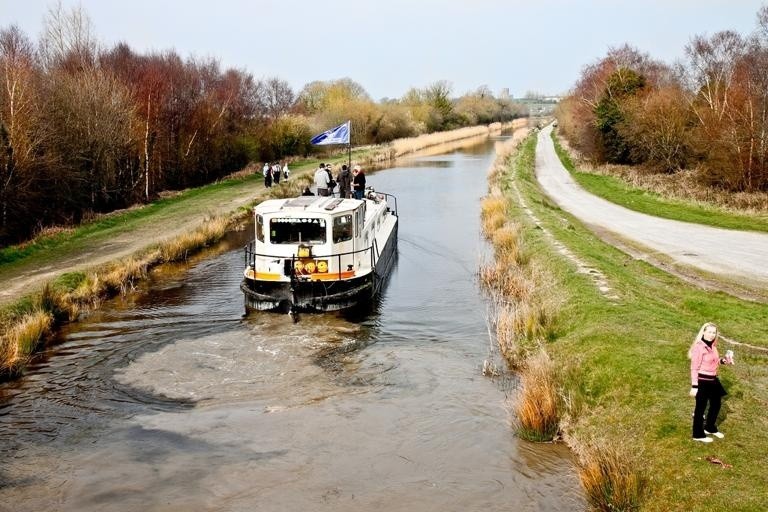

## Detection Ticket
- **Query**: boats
[240,120,398,315]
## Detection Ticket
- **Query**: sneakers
[704,429,724,439]
[693,436,713,443]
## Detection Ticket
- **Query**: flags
[310,121,350,145]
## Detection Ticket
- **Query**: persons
[273,161,282,184]
[263,161,273,188]
[302,185,315,197]
[314,162,366,199]
[690,321,737,446]
[283,161,290,182]
[270,161,276,180]
